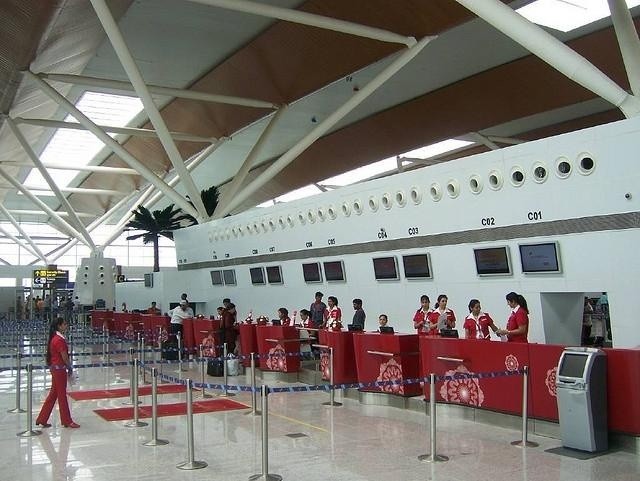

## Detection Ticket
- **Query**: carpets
[93,396,252,423]
[66,383,201,401]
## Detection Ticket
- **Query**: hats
[179,300,188,305]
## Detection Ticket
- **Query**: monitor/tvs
[518,241,562,275]
[348,324,363,331]
[556,350,593,383]
[472,245,513,277]
[372,255,400,281]
[266,265,284,285]
[271,319,281,325]
[323,259,347,284]
[210,270,224,285]
[210,316,214,319]
[144,274,154,288]
[440,328,459,338]
[402,252,433,280]
[379,326,393,332]
[301,261,324,284]
[249,266,266,286]
[223,269,237,285]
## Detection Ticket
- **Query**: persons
[34,318,81,429]
[146,293,366,361]
[413,295,435,336]
[376,314,391,332]
[495,292,529,343]
[579,292,613,349]
[34,427,73,481]
[16,291,82,326]
[428,294,456,336]
[462,299,504,341]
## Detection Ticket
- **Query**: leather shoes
[64,422,80,428]
[36,421,51,428]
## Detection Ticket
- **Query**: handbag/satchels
[227,353,239,376]
[207,359,223,376]
[161,340,184,360]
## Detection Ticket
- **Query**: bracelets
[494,329,499,335]
[507,330,511,335]
